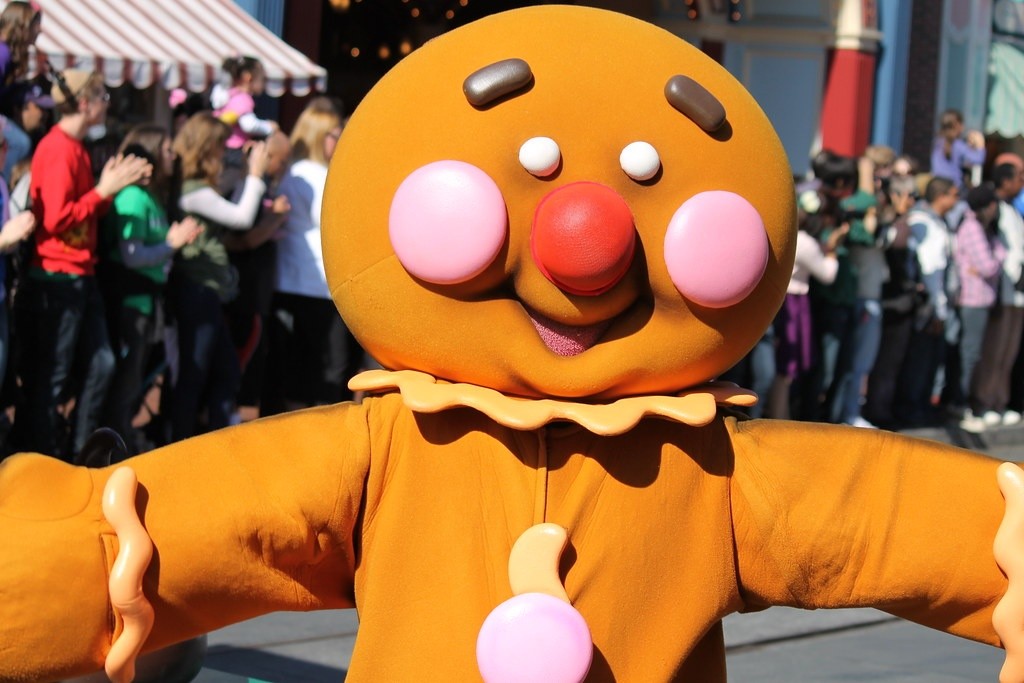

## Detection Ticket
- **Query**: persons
[761,109,1024,434]
[0,0,349,463]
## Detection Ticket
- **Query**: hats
[51,67,90,104]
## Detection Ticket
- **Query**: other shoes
[851,417,877,430]
[1002,409,1021,426]
[957,409,986,433]
[980,410,1000,425]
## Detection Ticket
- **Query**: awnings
[985,39,1024,137]
[0,0,328,98]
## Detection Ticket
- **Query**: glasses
[101,91,112,104]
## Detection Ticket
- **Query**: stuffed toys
[0,4,1024,683]
